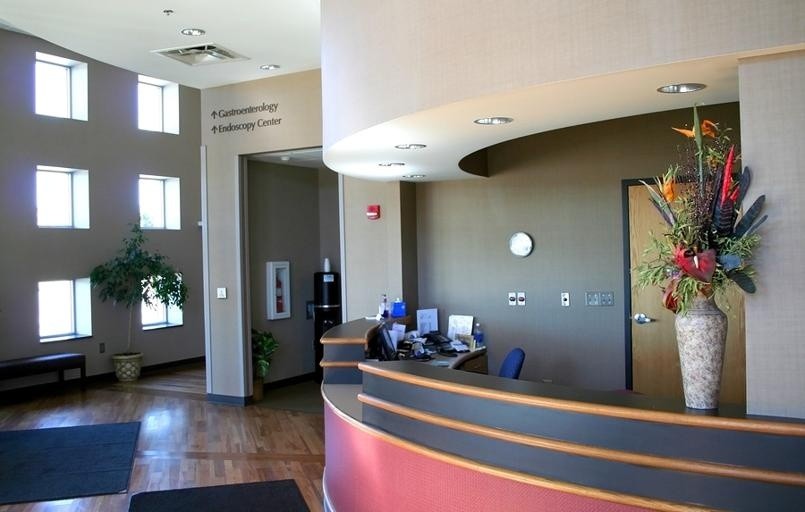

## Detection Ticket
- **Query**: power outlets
[216,287,226,299]
[600,291,613,305]
[586,292,600,306]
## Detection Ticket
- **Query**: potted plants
[90,223,189,382]
[250,326,277,401]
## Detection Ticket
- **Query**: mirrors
[510,233,533,257]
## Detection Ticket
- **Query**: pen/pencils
[383,293,387,312]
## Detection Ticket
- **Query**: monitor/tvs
[376,323,397,360]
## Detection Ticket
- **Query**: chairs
[500,348,525,381]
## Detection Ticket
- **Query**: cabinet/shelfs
[321,319,805,510]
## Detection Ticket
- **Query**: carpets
[128,480,310,512]
[0,421,141,504]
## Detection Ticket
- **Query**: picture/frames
[413,308,441,335]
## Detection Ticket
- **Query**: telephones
[450,340,470,353]
[423,332,459,358]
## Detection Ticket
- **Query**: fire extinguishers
[276,271,283,312]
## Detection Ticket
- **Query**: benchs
[0,352,85,406]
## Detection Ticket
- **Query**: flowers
[631,103,769,311]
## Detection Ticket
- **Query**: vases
[677,284,727,407]
